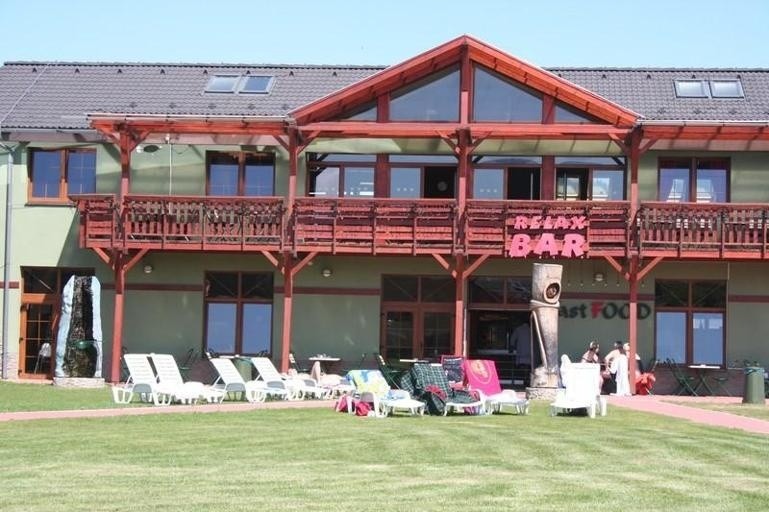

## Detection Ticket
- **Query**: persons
[621,342,644,374]
[510,315,538,390]
[599,340,627,395]
[579,340,600,363]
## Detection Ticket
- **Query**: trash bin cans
[742,367,765,405]
[233,357,252,382]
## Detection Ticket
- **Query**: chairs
[331,347,606,418]
[643,357,740,395]
[111,349,344,407]
